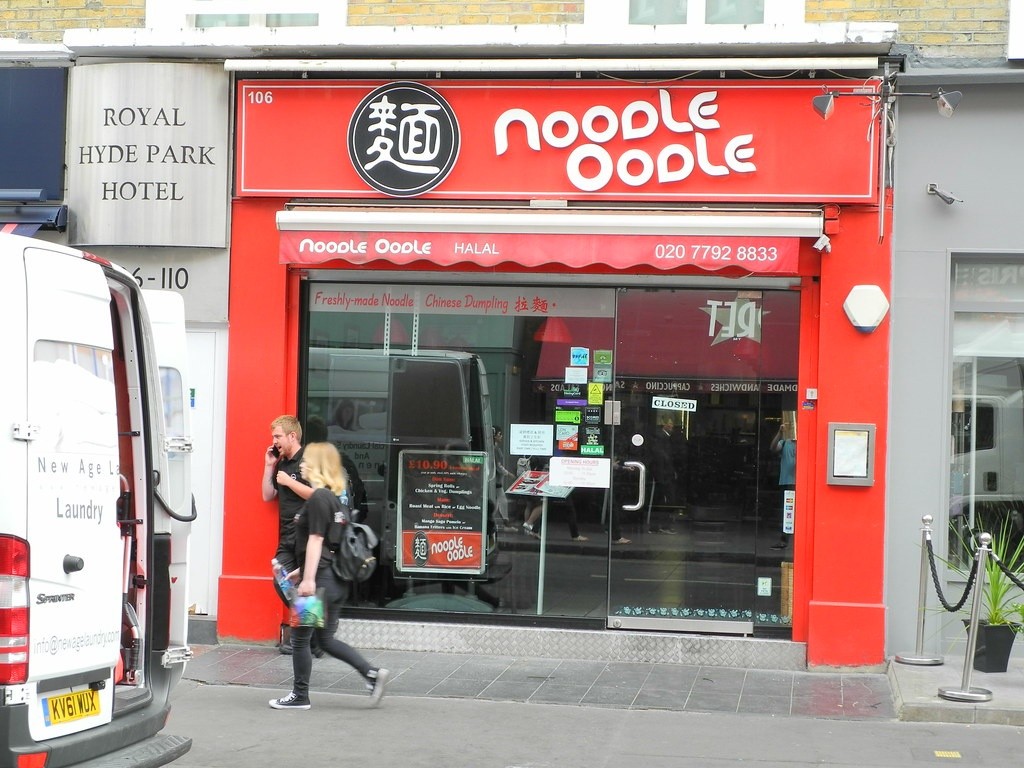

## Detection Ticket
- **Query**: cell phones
[271,445,280,458]
[785,423,794,431]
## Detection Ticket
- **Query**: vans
[307,342,513,598]
[0,230,202,768]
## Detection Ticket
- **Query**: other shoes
[526,529,541,540]
[611,537,632,545]
[648,528,661,534]
[522,522,538,533]
[573,534,590,542]
[659,528,678,535]
[769,543,789,550]
[278,638,293,655]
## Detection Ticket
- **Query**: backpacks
[331,498,380,584]
[517,455,533,477]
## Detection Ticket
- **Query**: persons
[267,442,389,709]
[261,416,332,658]
[304,400,357,442]
[442,414,796,610]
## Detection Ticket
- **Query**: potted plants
[919,511,1024,674]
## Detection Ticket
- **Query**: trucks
[952,389,1024,565]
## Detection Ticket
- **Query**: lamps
[843,284,890,332]
[892,87,962,118]
[813,92,882,119]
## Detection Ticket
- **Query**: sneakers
[269,691,311,709]
[366,668,391,709]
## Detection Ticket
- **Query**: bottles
[271,558,296,601]
[339,490,348,505]
